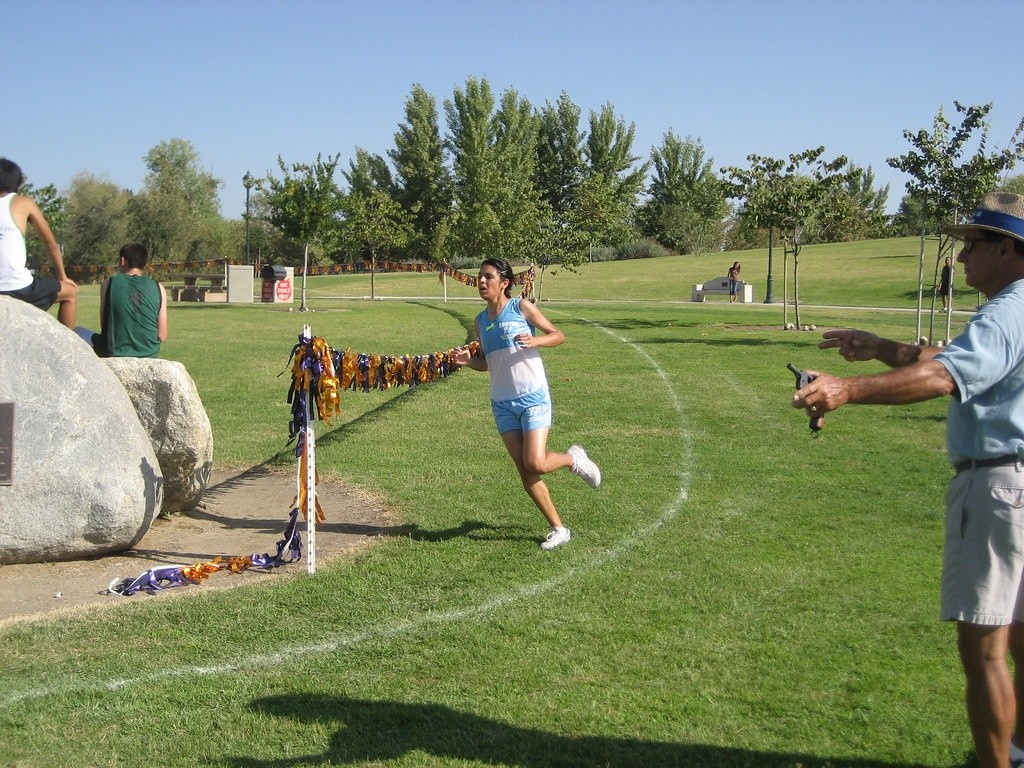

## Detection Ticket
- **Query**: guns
[787,363,826,432]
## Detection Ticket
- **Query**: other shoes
[939,308,948,313]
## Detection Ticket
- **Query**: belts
[956,455,1024,472]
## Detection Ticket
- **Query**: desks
[166,273,225,301]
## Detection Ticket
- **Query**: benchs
[164,286,227,301]
[692,277,753,303]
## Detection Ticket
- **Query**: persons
[0,158,79,331]
[72,242,167,358]
[449,256,601,549]
[791,192,1024,768]
[938,257,957,314]
[727,262,740,303]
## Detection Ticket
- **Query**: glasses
[964,236,1003,251]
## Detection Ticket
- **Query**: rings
[810,403,817,414]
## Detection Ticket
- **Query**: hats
[944,192,1024,242]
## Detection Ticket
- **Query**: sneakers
[540,528,570,551]
[567,444,601,489]
